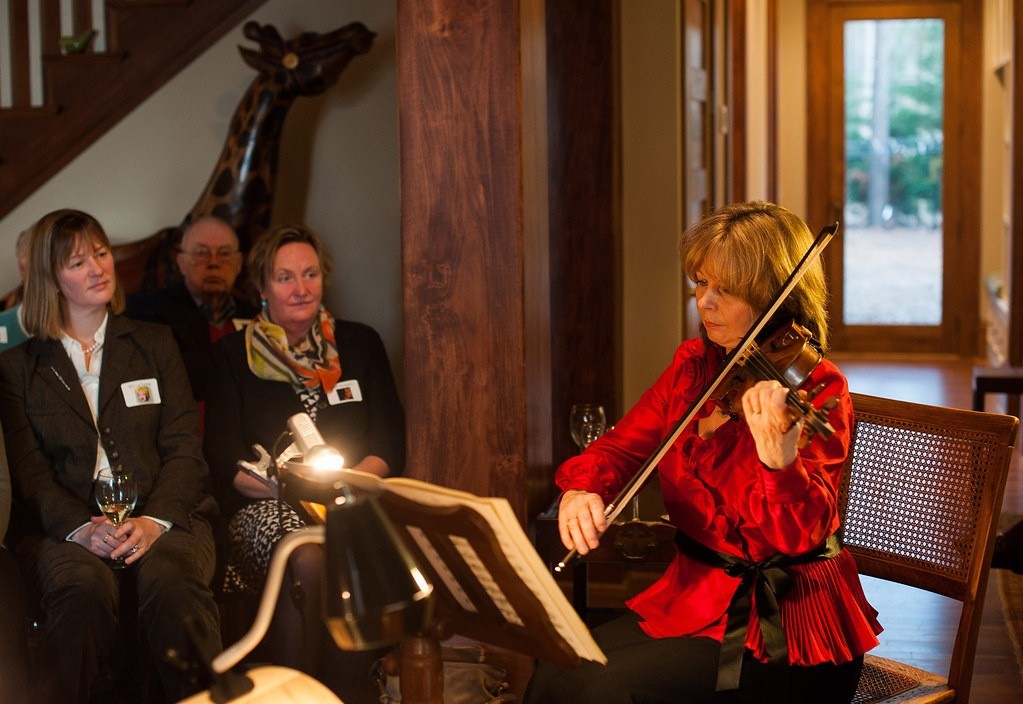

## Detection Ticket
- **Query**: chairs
[972,365,1023,434]
[838,393,1021,704]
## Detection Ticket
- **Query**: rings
[131,545,138,552]
[103,535,108,543]
[567,517,577,522]
[753,411,760,414]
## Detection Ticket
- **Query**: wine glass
[95,466,138,570]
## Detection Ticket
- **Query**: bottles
[398,620,446,704]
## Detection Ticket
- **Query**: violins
[708,310,841,443]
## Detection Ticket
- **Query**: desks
[533,478,672,620]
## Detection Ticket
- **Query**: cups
[570,403,606,454]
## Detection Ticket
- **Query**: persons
[128,216,260,444]
[205,222,407,680]
[0,209,222,704]
[527,203,885,704]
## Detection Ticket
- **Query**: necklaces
[84,343,98,353]
[717,410,727,417]
